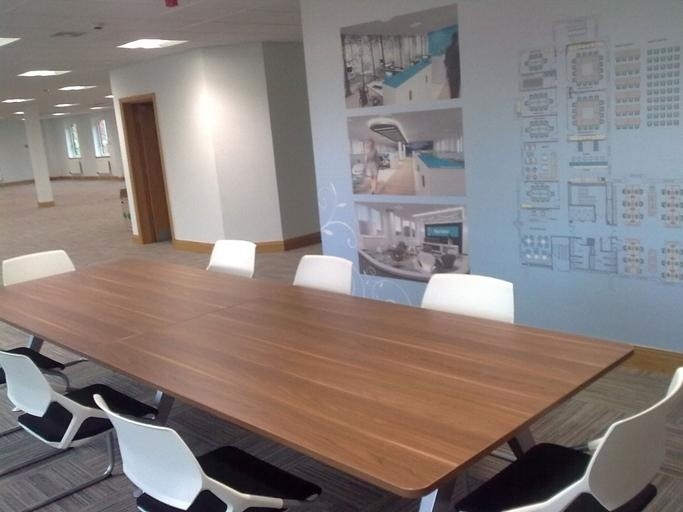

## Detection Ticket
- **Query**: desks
[1,256,633,511]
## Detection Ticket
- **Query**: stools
[1,347,73,438]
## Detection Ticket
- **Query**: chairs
[204,239,256,280]
[420,273,515,326]
[2,249,76,286]
[451,366,682,511]
[2,351,157,511]
[291,254,352,297]
[90,392,322,511]
